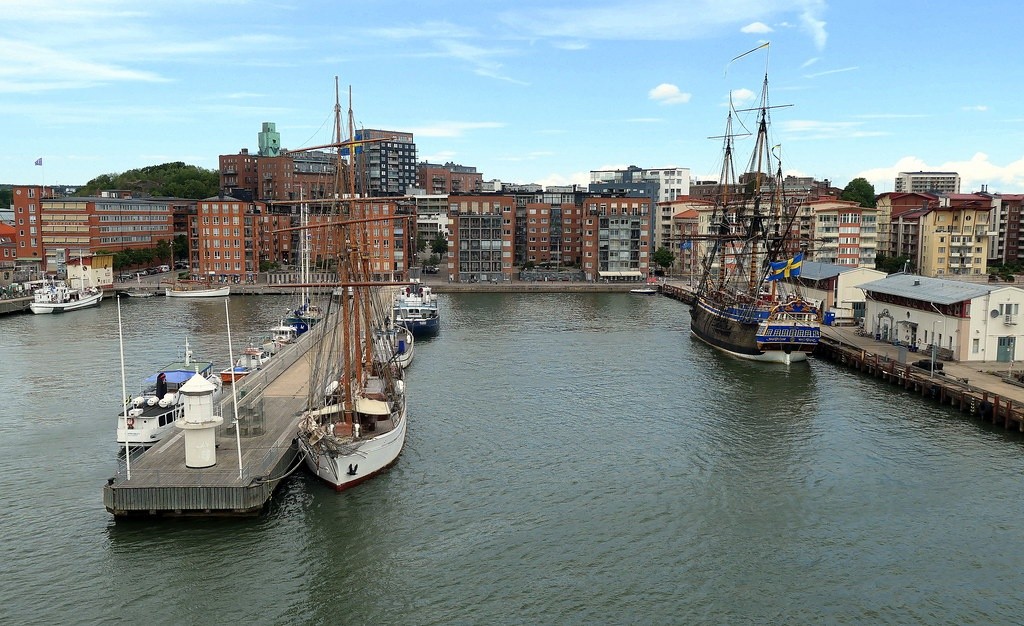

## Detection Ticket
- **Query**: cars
[113,264,170,280]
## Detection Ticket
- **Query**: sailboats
[261,76,426,493]
[688,39,825,367]
[164,241,231,298]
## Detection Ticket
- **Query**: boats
[384,276,441,336]
[27,249,105,315]
[630,287,657,294]
[219,185,353,386]
[121,289,154,298]
[360,269,416,369]
[115,338,224,447]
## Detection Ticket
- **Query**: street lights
[864,292,878,341]
[903,259,911,272]
[838,298,851,346]
[930,320,943,378]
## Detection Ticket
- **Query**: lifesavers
[978,400,992,416]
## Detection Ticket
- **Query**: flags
[35,158,42,166]
[767,252,804,281]
